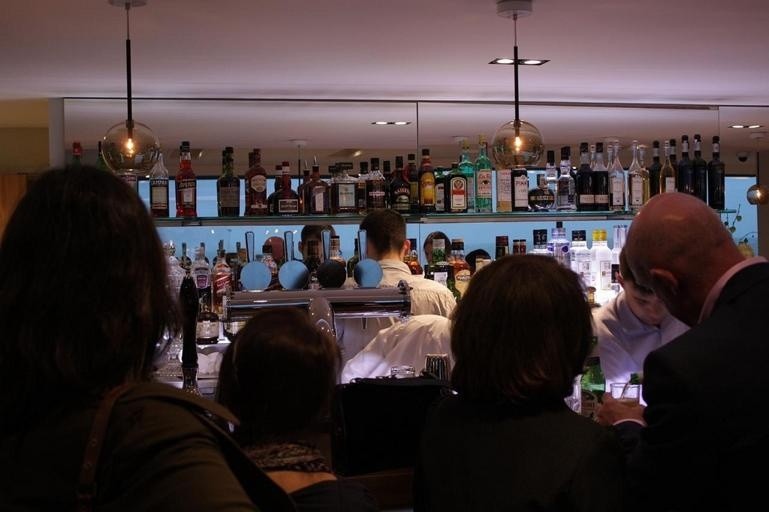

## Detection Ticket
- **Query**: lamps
[493,0,543,168]
[102,0,159,177]
[747,132,769,205]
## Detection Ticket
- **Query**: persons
[594,192,769,511]
[0,162,297,512]
[597,244,692,407]
[211,307,377,510]
[413,254,628,510]
[296,208,491,385]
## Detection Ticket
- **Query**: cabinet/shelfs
[138,209,740,397]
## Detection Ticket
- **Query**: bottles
[458,141,493,214]
[406,148,433,213]
[175,140,198,216]
[527,173,555,211]
[268,161,301,215]
[149,134,169,217]
[244,147,268,217]
[298,156,411,215]
[150,221,633,346]
[496,154,528,212]
[216,147,241,217]
[581,337,607,421]
[96,139,109,170]
[72,139,84,163]
[433,162,468,213]
[545,132,724,213]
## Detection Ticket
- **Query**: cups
[391,364,415,379]
[610,382,642,407]
